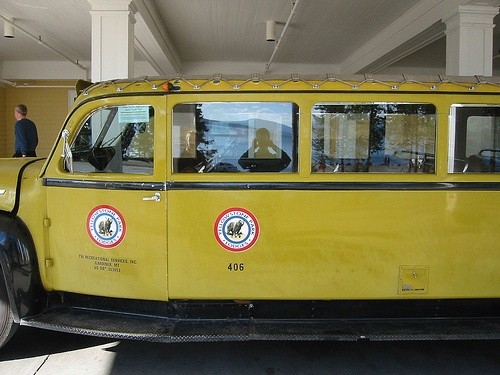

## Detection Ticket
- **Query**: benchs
[309,148,500,173]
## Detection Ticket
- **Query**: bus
[0,74,500,350]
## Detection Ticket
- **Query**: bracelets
[22,154,27,156]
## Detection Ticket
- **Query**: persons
[177,131,211,173]
[238,129,290,172]
[10,103,39,159]
